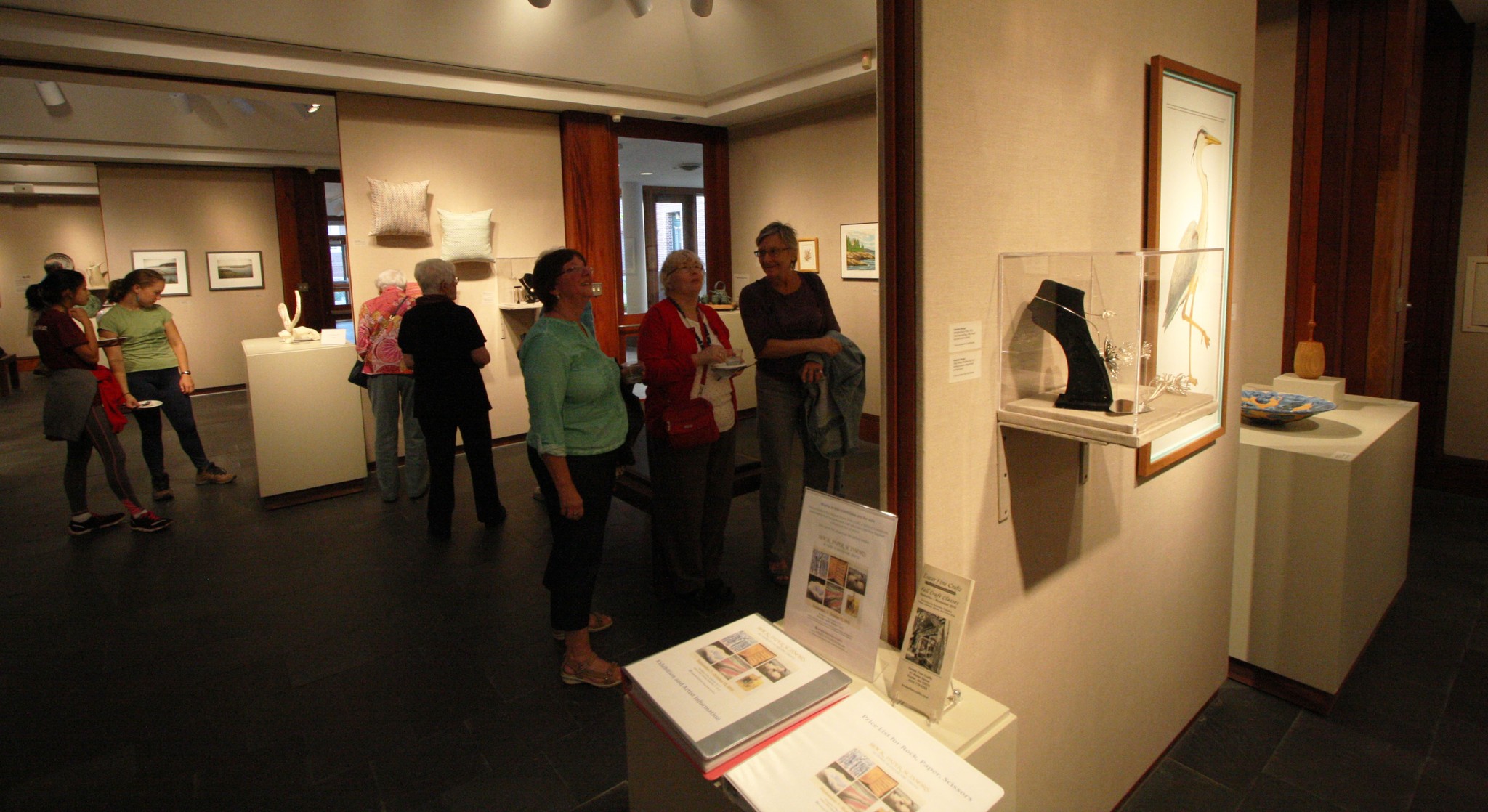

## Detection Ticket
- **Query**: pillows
[435,208,496,265]
[367,176,432,238]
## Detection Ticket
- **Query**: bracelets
[181,371,191,375]
[124,392,130,395]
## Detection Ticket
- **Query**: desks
[610,446,765,517]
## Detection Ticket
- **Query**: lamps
[303,103,321,113]
[625,0,653,18]
[690,0,714,17]
[171,92,193,114]
[528,0,550,8]
[36,80,64,106]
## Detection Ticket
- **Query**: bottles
[513,286,522,304]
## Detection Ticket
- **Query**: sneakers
[196,460,236,485]
[151,484,174,500]
[128,510,172,532]
[70,511,125,535]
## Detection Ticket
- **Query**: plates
[97,337,128,344]
[138,400,163,408]
[43,253,74,274]
[713,362,747,370]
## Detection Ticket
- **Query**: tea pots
[709,281,727,301]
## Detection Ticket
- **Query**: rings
[720,353,722,358]
[574,513,578,515]
[814,369,819,373]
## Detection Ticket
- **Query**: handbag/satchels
[662,397,720,446]
[347,357,369,389]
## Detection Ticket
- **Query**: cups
[621,361,646,383]
[1293,340,1325,380]
[711,295,721,304]
[701,295,709,304]
[721,296,732,304]
[724,349,742,365]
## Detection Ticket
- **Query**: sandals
[553,611,613,641]
[560,653,623,688]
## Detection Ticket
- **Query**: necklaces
[669,297,711,351]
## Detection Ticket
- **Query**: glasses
[558,266,594,278]
[754,248,790,258]
[450,277,459,284]
[666,263,703,277]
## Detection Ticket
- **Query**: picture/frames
[798,237,819,273]
[131,249,191,297]
[205,250,265,291]
[1137,56,1243,479]
[840,223,880,281]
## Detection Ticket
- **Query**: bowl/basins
[1240,390,1337,427]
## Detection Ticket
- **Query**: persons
[398,258,507,550]
[96,268,237,502]
[515,248,648,687]
[636,250,745,608]
[25,260,174,535]
[357,270,430,504]
[739,221,843,585]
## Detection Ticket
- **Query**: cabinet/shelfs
[1226,386,1421,713]
[240,336,369,509]
[626,616,1019,812]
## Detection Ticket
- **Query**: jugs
[86,262,106,287]
